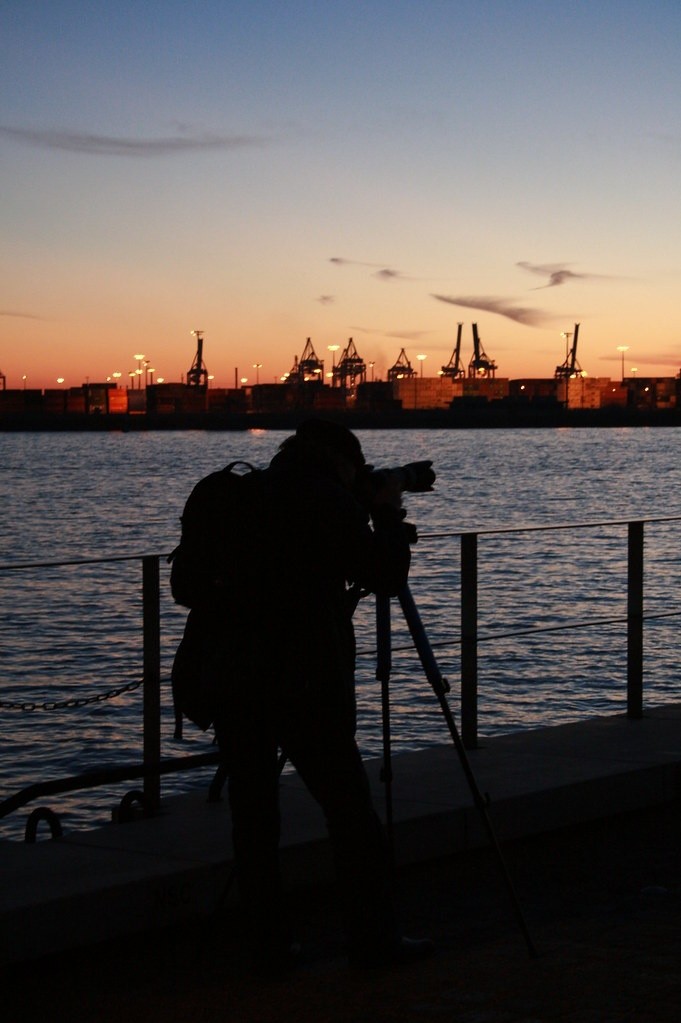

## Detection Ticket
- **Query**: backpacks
[167,462,318,783]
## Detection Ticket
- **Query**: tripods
[196,516,543,960]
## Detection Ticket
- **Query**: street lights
[112,371,121,383]
[253,364,262,384]
[22,375,27,389]
[147,368,155,384]
[368,362,375,382]
[134,354,145,389]
[143,361,150,385]
[617,346,630,380]
[328,345,339,366]
[561,332,573,367]
[129,372,136,389]
[417,354,426,378]
[631,367,638,378]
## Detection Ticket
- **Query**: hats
[297,418,357,465]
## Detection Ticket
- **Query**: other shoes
[349,937,433,968]
[257,959,297,984]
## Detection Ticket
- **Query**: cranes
[296,336,325,386]
[467,322,498,379]
[186,337,210,390]
[330,336,367,395]
[553,322,582,380]
[386,348,419,383]
[439,321,466,379]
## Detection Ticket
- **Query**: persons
[173,425,436,962]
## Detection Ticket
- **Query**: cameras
[360,459,437,518]
